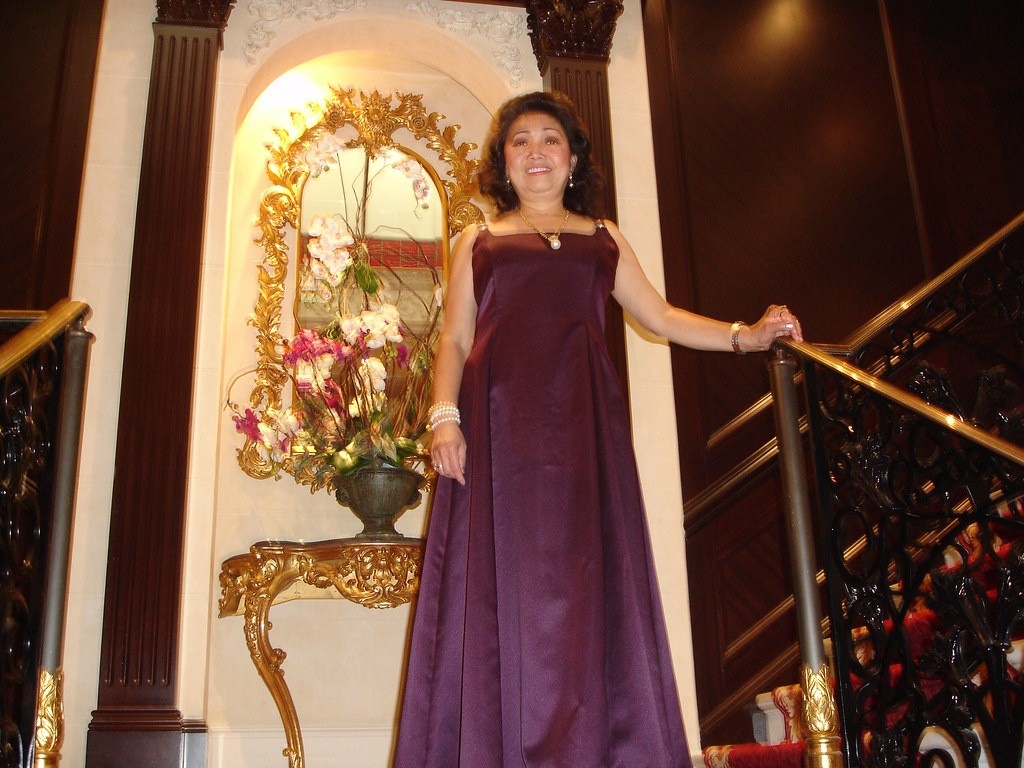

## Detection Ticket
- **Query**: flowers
[224,124,448,482]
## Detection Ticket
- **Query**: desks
[218,540,424,768]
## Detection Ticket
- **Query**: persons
[387,91,803,768]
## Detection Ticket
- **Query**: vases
[335,468,429,537]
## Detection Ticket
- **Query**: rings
[779,305,799,323]
[434,464,442,470]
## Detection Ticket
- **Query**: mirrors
[235,83,488,495]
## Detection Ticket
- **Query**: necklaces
[517,206,569,249]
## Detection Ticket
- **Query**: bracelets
[427,402,461,428]
[731,321,749,355]
